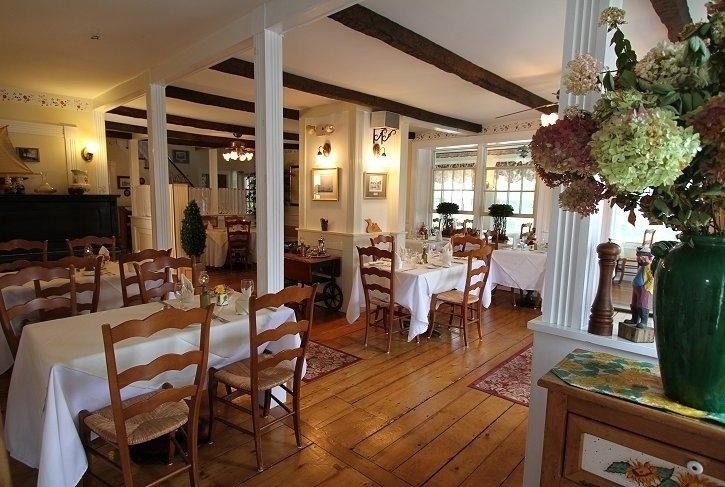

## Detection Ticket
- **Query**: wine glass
[241,279,255,295]
[198,271,210,285]
[173,283,190,302]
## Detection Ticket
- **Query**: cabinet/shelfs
[531,345,724,487]
[0,193,122,253]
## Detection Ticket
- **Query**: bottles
[34,167,55,193]
[586,238,619,337]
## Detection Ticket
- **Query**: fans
[493,89,559,119]
[194,130,257,152]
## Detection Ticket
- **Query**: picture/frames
[362,170,390,200]
[308,166,343,204]
[172,149,191,164]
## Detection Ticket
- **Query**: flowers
[525,0,725,236]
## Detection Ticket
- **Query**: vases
[0,168,90,194]
[648,233,725,411]
[116,173,130,191]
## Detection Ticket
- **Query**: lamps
[222,149,254,162]
[539,110,558,126]
[79,144,97,163]
[371,143,390,161]
[315,141,332,159]
[305,120,336,137]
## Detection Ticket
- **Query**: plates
[188,320,215,329]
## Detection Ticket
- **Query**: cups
[320,218,329,232]
[421,247,428,264]
[419,221,428,240]
